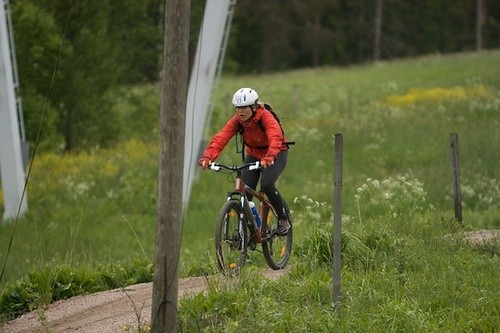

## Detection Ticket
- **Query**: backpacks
[235,104,284,150]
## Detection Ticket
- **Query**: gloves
[261,156,274,168]
[198,158,209,170]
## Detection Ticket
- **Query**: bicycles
[206,160,293,278]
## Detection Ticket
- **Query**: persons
[199,88,291,236]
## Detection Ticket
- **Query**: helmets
[232,88,259,107]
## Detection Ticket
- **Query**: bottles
[248,202,261,227]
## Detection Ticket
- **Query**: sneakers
[273,218,291,235]
